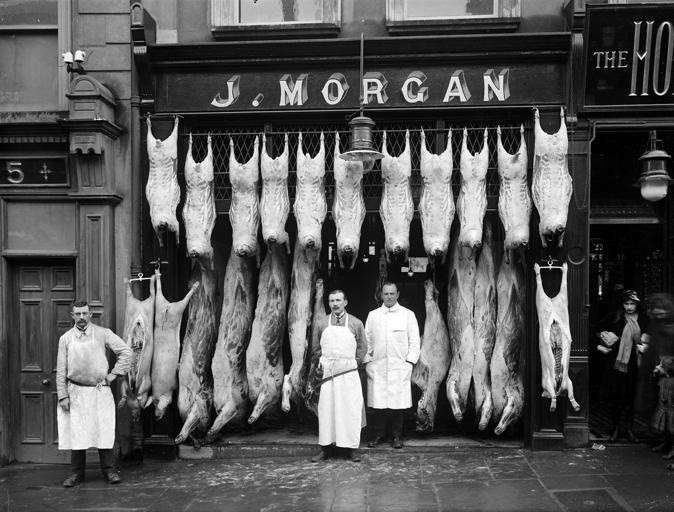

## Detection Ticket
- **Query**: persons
[596,289,649,445]
[645,292,673,460]
[311,290,366,462]
[363,282,420,450]
[55,301,133,488]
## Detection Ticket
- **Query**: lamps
[338,28,387,176]
[635,131,672,202]
[62,50,87,74]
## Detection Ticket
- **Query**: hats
[624,289,640,301]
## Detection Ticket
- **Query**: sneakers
[347,451,360,461]
[368,438,383,446]
[63,474,83,486]
[107,471,121,483]
[393,438,402,448]
[312,451,327,461]
